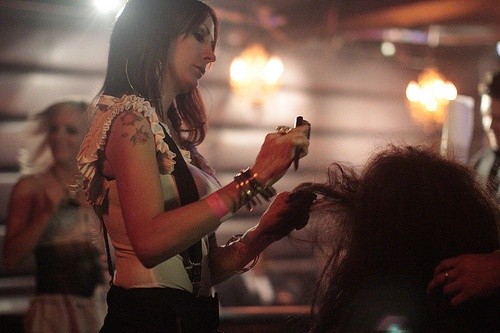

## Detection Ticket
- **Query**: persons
[85,0,315,333]
[290,69,500,333]
[0,99,134,333]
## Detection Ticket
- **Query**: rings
[444,272,449,281]
[276,126,292,134]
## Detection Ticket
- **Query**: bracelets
[201,169,277,221]
[223,234,260,274]
